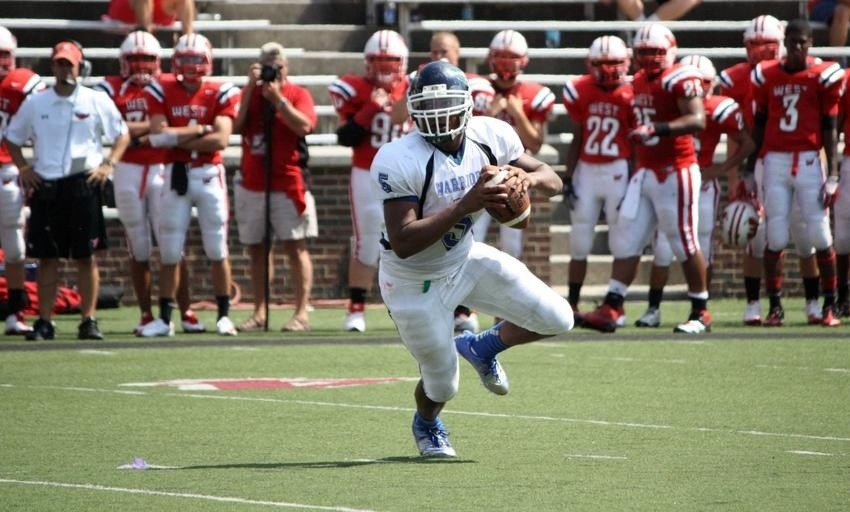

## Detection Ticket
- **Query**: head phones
[66,38,92,77]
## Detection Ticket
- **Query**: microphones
[60,78,86,87]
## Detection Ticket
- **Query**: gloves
[819,175,841,209]
[735,172,758,201]
[561,176,578,210]
[627,123,655,145]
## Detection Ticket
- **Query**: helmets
[120,31,162,78]
[406,62,473,145]
[743,15,784,66]
[364,30,409,86]
[173,34,213,85]
[632,23,678,75]
[680,55,716,102]
[486,30,529,82]
[0,25,17,76]
[721,201,758,248]
[589,35,631,88]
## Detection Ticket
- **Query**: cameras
[259,65,278,82]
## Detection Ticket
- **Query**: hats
[52,40,83,65]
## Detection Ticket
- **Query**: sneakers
[78,315,103,339]
[762,306,786,326]
[24,317,55,342]
[215,316,238,336]
[281,317,311,332]
[133,312,153,333]
[180,309,205,332]
[454,333,510,396]
[805,298,823,324]
[455,313,480,334]
[343,303,366,333]
[412,413,456,459]
[4,319,30,334]
[634,308,661,328]
[235,313,265,332]
[821,296,841,326]
[135,318,175,338]
[834,297,850,318]
[743,300,762,324]
[573,304,619,332]
[616,307,625,327]
[673,309,713,334]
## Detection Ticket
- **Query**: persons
[461,29,557,332]
[92,30,207,338]
[616,1,704,21]
[232,41,319,333]
[106,0,196,35]
[635,55,757,329]
[829,0,849,46]
[561,34,636,327]
[833,66,850,324]
[326,30,414,332]
[743,17,850,328]
[390,31,497,331]
[137,32,244,339]
[2,40,131,341]
[573,23,713,334]
[369,60,576,461]
[719,13,824,325]
[0,25,46,336]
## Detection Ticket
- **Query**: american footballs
[481,165,531,230]
